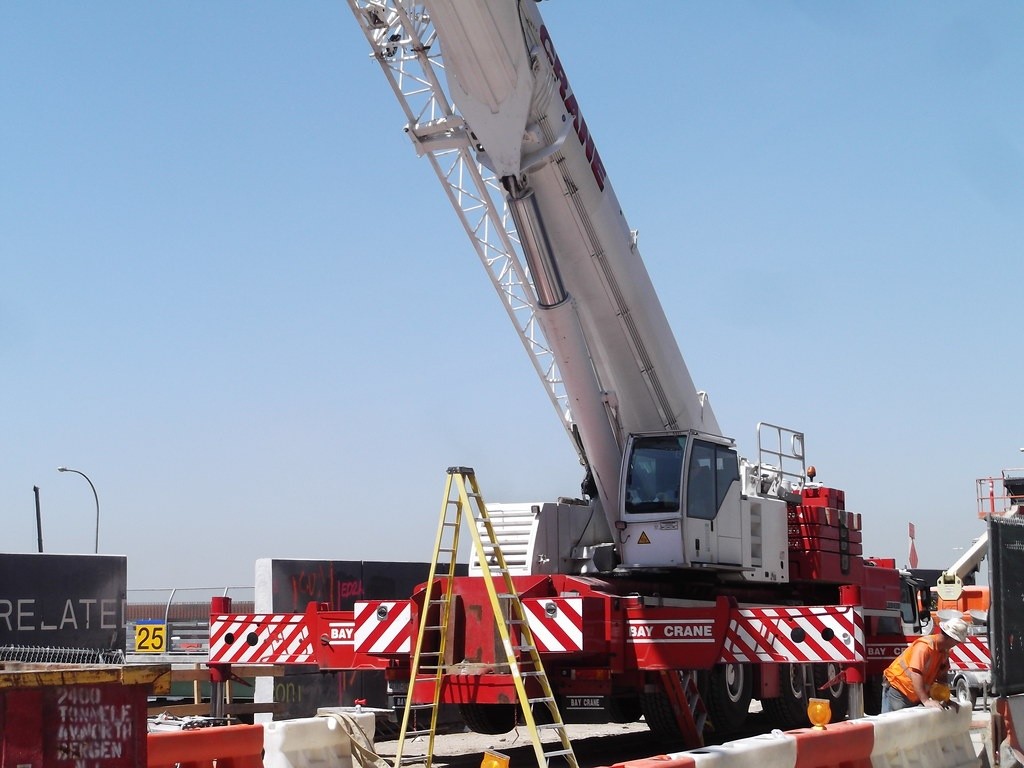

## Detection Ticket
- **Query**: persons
[881,616,967,714]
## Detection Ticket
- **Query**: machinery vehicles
[203,2,918,752]
[916,472,1024,712]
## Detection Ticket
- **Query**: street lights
[58,466,100,555]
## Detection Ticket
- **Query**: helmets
[939,618,968,643]
